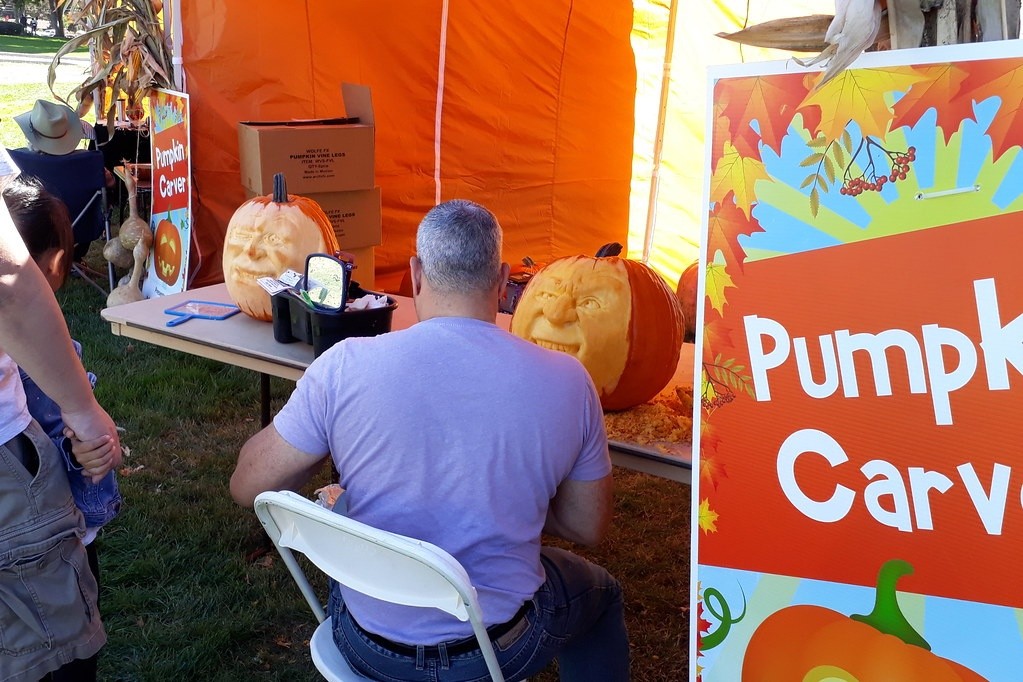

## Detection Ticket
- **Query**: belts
[347,600,537,658]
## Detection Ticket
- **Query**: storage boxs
[238,79,383,197]
[331,246,378,292]
[288,184,382,255]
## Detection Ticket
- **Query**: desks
[101,274,698,487]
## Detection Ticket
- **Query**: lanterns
[507,240,686,414]
[222,173,342,323]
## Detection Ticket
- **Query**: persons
[1,178,121,612]
[1,188,119,682]
[4,12,41,38]
[228,197,631,682]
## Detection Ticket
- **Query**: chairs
[248,488,506,682]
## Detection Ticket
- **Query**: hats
[13,99,84,155]
